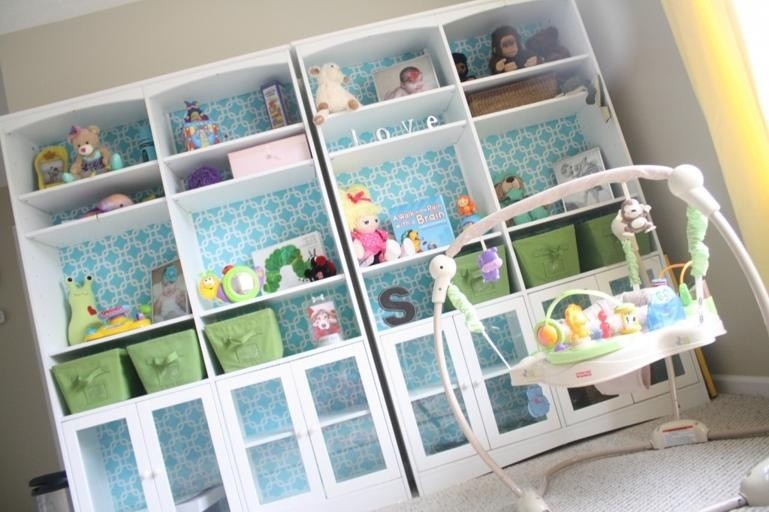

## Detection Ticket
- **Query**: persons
[48,166,59,184]
[384,66,428,101]
[153,264,188,323]
[402,230,420,254]
[561,156,605,208]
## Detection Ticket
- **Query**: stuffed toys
[494,173,549,227]
[188,165,221,189]
[307,61,361,126]
[559,73,597,104]
[452,52,476,82]
[621,199,656,234]
[488,26,541,73]
[67,274,104,346]
[337,184,416,269]
[477,247,502,284]
[198,264,266,310]
[82,192,135,217]
[181,100,221,152]
[456,195,482,233]
[525,27,572,65]
[304,255,337,282]
[61,124,123,183]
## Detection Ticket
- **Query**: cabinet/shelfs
[292,2,712,497]
[0,46,413,511]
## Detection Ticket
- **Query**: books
[251,231,327,296]
[551,146,615,213]
[387,193,455,255]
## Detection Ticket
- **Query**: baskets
[467,69,558,118]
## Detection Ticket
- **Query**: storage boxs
[51,347,128,414]
[583,211,651,268]
[203,307,285,374]
[126,328,204,394]
[511,225,582,288]
[453,243,511,305]
